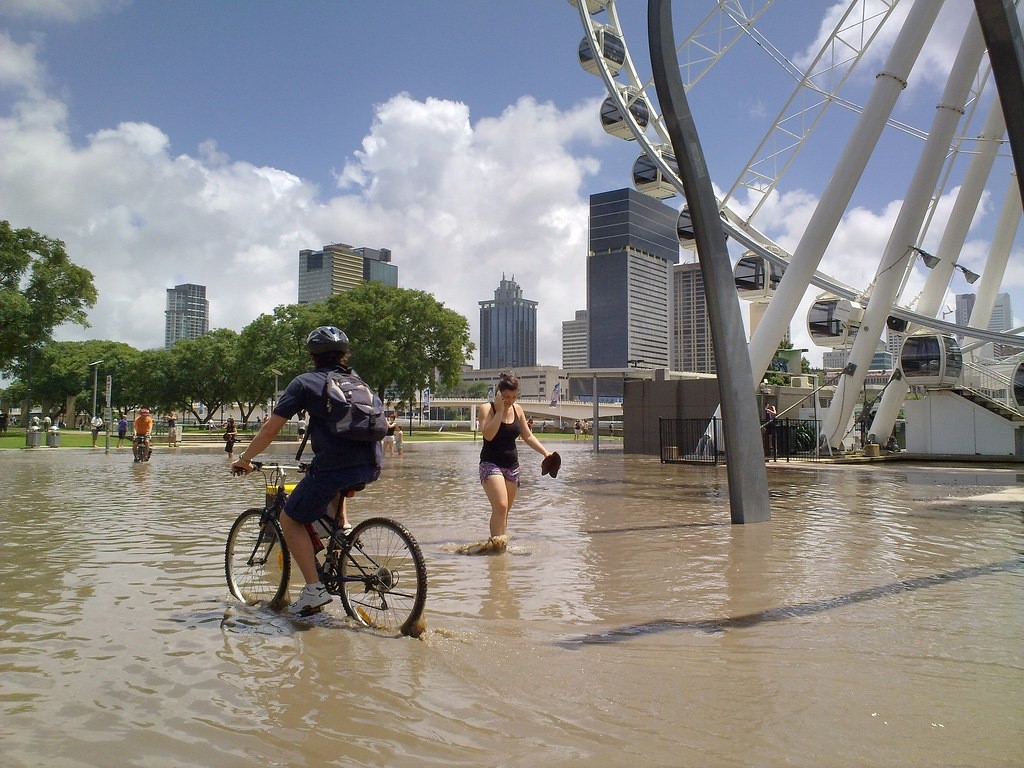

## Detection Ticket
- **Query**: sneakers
[289,581,334,614]
[325,524,354,545]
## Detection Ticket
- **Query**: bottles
[305,523,325,554]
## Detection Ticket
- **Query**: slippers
[542,452,561,479]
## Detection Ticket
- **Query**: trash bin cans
[26,426,41,446]
[46,426,61,446]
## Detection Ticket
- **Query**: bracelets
[239,451,251,464]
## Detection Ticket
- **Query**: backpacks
[316,369,386,441]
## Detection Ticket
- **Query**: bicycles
[223,459,427,635]
[132,434,154,462]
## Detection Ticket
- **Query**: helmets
[304,326,348,360]
[227,417,234,425]
[140,409,150,416]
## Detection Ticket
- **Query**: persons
[90,413,103,447]
[231,326,382,615]
[527,419,533,430]
[44,415,51,432]
[298,417,306,439]
[0,409,8,437]
[132,408,153,462]
[256,413,269,423]
[225,414,237,458]
[765,402,776,449]
[477,372,552,538]
[32,415,39,426]
[383,415,403,457]
[117,415,127,448]
[574,419,589,440]
[167,411,178,447]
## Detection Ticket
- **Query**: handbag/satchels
[266,485,352,585]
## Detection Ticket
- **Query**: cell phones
[496,390,502,397]
